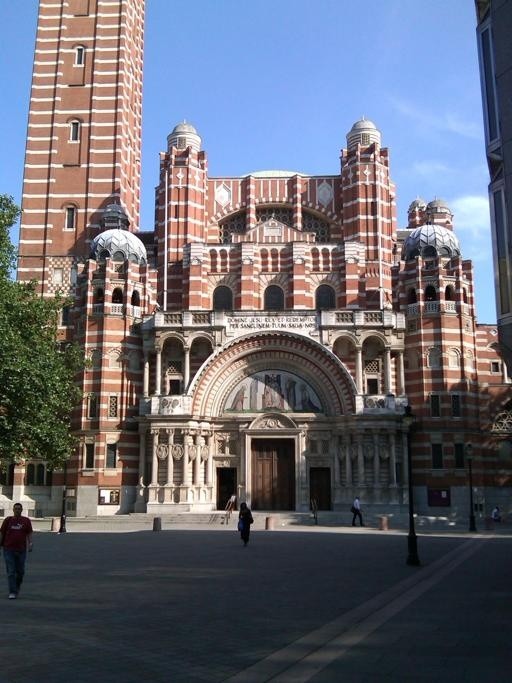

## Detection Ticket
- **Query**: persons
[224,374,318,412]
[0,502,36,600]
[491,505,502,522]
[237,502,254,547]
[350,495,364,526]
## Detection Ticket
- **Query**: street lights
[399,406,421,564]
[60,448,71,532]
[466,444,477,531]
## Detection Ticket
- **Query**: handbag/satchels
[237,518,244,532]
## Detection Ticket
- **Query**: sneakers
[8,592,18,599]
